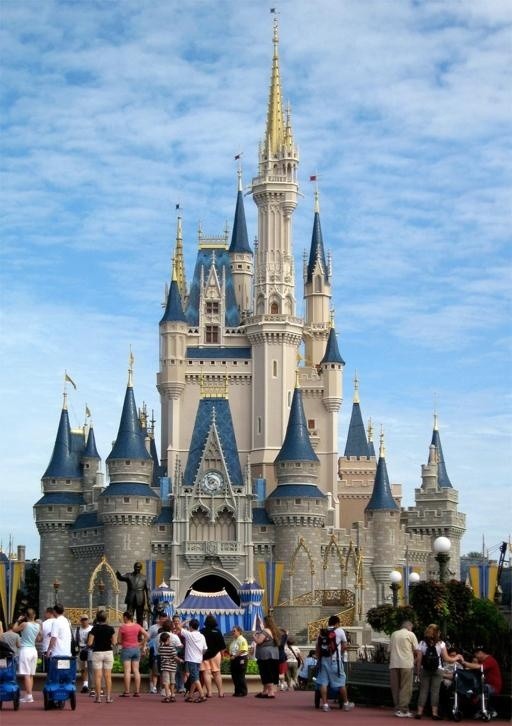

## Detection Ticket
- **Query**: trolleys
[308,651,346,709]
[0,653,22,711]
[448,662,490,723]
[42,656,79,712]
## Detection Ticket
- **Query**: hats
[79,613,89,621]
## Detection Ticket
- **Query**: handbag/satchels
[71,638,80,657]
[296,656,304,668]
[79,649,89,661]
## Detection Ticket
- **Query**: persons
[199,616,226,698]
[253,615,281,698]
[1,604,189,702]
[317,616,355,712]
[251,628,318,691]
[178,619,208,703]
[229,626,248,696]
[387,621,502,720]
[116,562,151,642]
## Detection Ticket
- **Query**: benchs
[311,660,444,714]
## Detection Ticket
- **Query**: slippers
[206,691,226,698]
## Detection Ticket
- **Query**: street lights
[53,579,61,607]
[98,579,106,612]
[407,571,420,585]
[389,571,402,608]
[433,537,452,584]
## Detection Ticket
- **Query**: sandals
[170,696,177,703]
[160,697,171,703]
[185,697,194,702]
[133,692,141,697]
[194,696,208,703]
[118,691,131,697]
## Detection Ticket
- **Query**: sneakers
[158,687,167,697]
[150,686,158,694]
[100,690,105,696]
[473,710,499,721]
[19,694,34,703]
[94,697,102,703]
[232,692,276,698]
[322,705,331,712]
[393,709,442,721]
[106,697,114,703]
[88,691,96,697]
[343,701,355,711]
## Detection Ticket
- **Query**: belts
[78,644,88,649]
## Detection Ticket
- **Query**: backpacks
[317,626,339,657]
[422,638,438,672]
